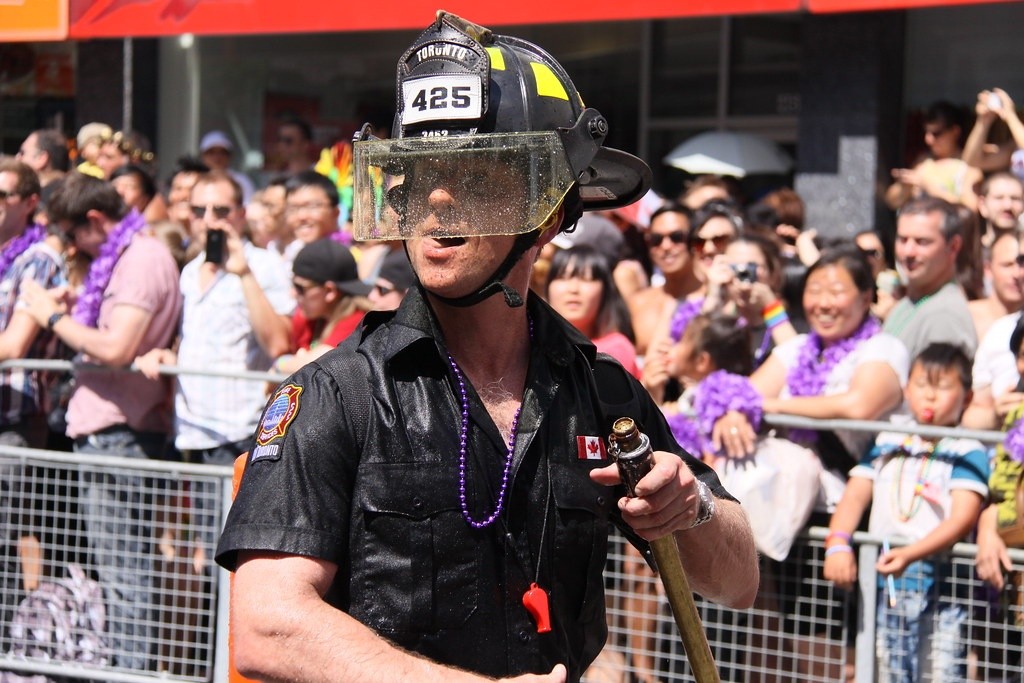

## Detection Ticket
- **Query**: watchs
[690,477,715,528]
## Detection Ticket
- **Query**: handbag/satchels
[712,429,823,561]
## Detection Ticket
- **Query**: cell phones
[988,94,1002,112]
[206,229,224,264]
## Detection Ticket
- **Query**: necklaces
[669,297,703,341]
[663,367,762,460]
[78,211,143,328]
[786,317,881,446]
[468,432,551,633]
[0,227,44,275]
[884,278,956,335]
[449,353,521,528]
[894,436,939,521]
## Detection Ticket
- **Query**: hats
[199,131,233,155]
[550,212,625,261]
[292,240,370,297]
[75,122,111,153]
[379,249,418,288]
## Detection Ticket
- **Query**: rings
[730,426,737,434]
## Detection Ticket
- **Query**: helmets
[350,9,654,243]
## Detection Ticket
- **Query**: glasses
[294,283,321,296]
[0,190,24,204]
[649,231,687,246]
[692,233,732,254]
[63,209,101,242]
[190,204,231,220]
[372,283,394,296]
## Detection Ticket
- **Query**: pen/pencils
[882,539,898,607]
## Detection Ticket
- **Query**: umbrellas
[665,131,791,179]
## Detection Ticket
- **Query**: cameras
[729,263,758,284]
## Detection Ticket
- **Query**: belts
[75,424,129,449]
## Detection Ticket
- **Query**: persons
[533,88,1024,683]
[0,114,417,683]
[215,8,758,683]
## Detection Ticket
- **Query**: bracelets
[824,533,850,544]
[825,545,852,557]
[761,301,789,329]
[275,355,291,372]
[49,313,64,330]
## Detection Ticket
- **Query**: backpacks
[0,565,111,683]
